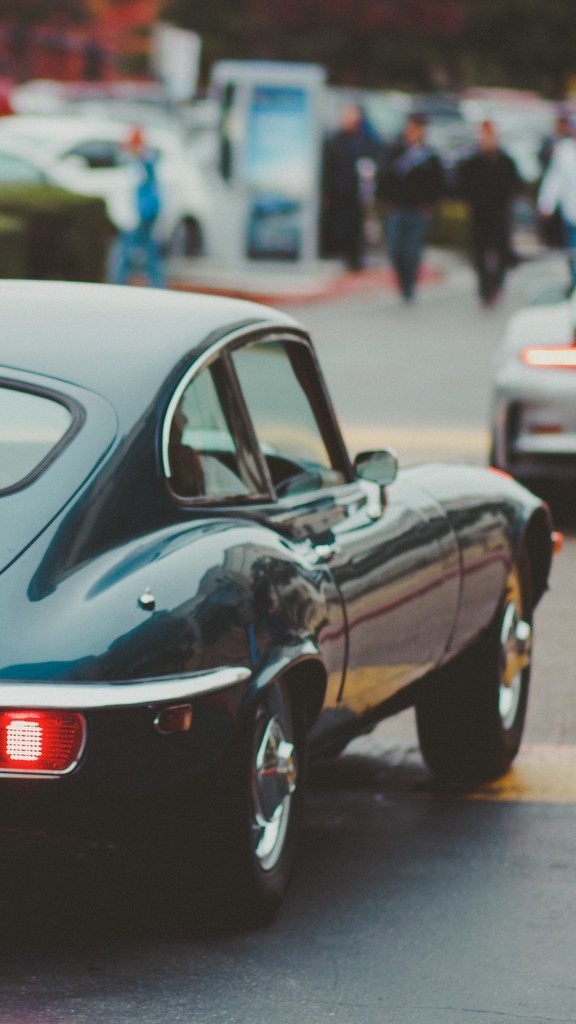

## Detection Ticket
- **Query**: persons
[318,105,576,308]
[105,129,172,289]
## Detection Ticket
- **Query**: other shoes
[477,277,504,308]
[563,272,576,300]
[399,293,414,308]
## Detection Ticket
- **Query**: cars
[0,275,568,934]
[0,69,564,272]
[485,289,576,535]
